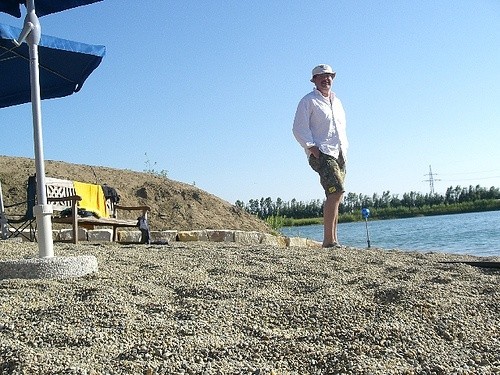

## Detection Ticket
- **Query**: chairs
[4,173,38,242]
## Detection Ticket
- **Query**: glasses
[315,74,332,79]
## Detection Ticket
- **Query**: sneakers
[322,242,340,250]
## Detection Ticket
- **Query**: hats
[309,64,336,82]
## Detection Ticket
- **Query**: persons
[292,64,348,247]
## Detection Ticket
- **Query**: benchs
[45,176,151,244]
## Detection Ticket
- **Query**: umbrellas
[1,23,107,111]
[1,0,114,254]
